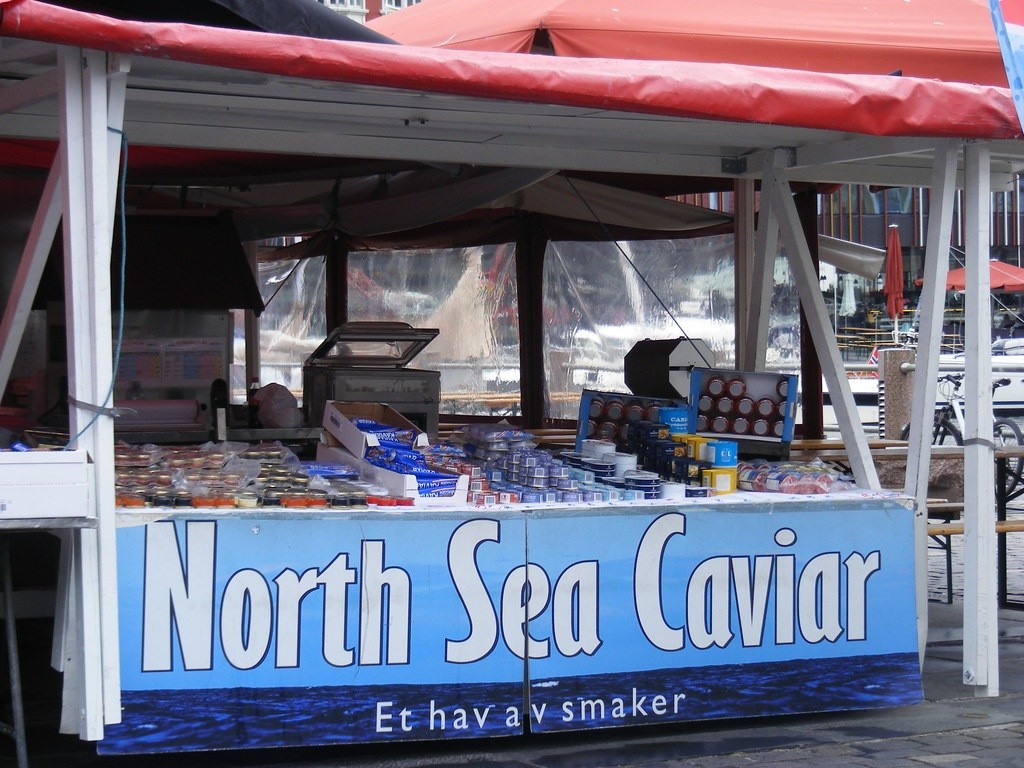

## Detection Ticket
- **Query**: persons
[998,314,1015,329]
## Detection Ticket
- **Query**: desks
[437,430,463,437]
[526,429,577,436]
[789,438,907,451]
[437,423,470,428]
[532,434,577,444]
[789,445,1024,611]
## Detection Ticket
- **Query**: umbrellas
[885,228,904,344]
[839,273,856,326]
[913,259,1024,294]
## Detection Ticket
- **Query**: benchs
[927,519,1024,537]
[927,498,964,513]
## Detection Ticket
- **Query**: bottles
[247,377,260,428]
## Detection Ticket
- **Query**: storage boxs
[316,444,358,468]
[359,460,470,507]
[686,365,799,442]
[575,387,689,452]
[321,400,430,460]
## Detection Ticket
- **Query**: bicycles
[899,372,1024,497]
